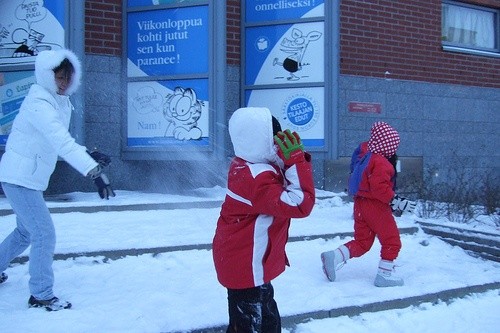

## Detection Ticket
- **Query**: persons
[0,49,116,312]
[321,121,416,287]
[212,107,316,333]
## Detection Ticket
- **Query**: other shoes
[28,294,72,312]
[0,272,8,284]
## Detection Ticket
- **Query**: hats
[272,116,282,136]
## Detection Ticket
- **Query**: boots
[375,260,404,287]
[321,245,350,282]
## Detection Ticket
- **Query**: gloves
[86,150,112,169]
[88,165,116,200]
[390,194,416,217]
[273,128,306,167]
